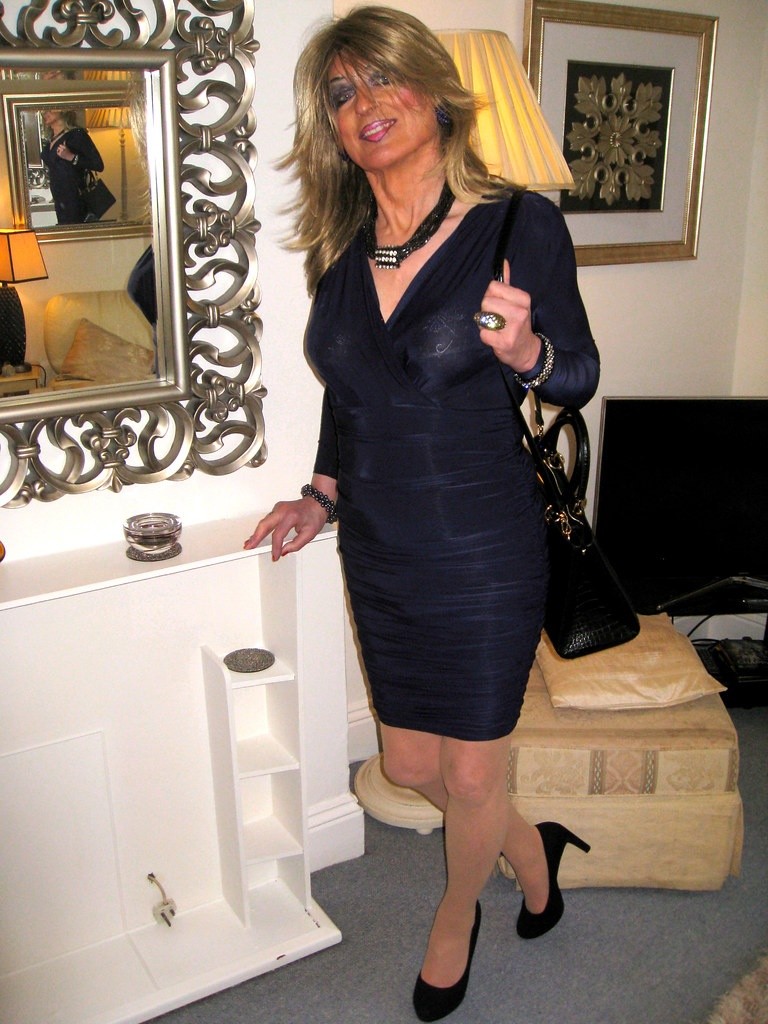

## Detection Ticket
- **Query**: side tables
[0,361,41,396]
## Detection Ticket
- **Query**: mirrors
[0,0,269,509]
[22,111,50,189]
[2,89,153,244]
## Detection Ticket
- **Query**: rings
[57,149,60,153]
[473,311,505,331]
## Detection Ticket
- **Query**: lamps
[431,28,578,192]
[0,228,49,378]
[86,108,132,224]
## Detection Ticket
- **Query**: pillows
[60,317,155,382]
[537,614,729,713]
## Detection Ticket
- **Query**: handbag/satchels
[79,170,117,221]
[529,404,640,661]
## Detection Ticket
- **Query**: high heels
[517,821,591,940]
[413,898,482,1023]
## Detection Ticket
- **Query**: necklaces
[48,127,68,143]
[363,176,455,269]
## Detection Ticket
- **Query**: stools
[498,664,755,890]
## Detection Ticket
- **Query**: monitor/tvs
[591,395,768,646]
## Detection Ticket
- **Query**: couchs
[42,290,158,389]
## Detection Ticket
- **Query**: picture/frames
[521,0,721,268]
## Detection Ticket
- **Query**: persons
[239,6,600,1024]
[40,105,104,224]
[128,245,158,374]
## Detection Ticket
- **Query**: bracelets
[301,484,338,524]
[512,333,555,390]
[73,154,79,165]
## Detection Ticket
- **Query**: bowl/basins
[123,511,183,556]
